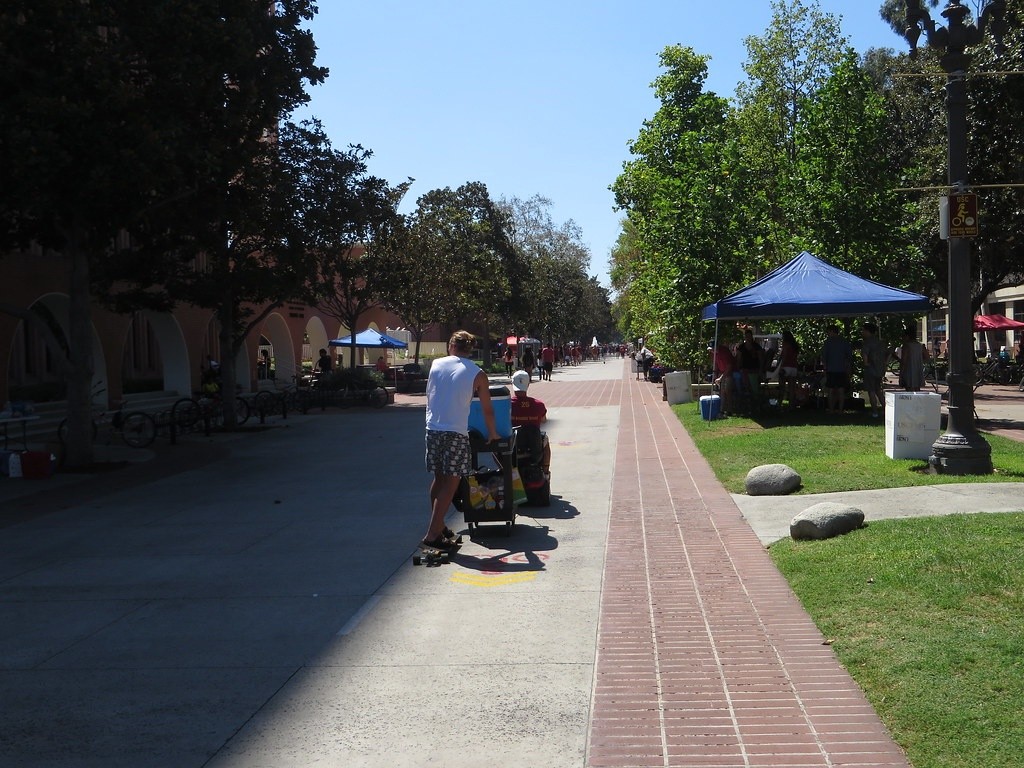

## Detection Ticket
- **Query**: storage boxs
[699,395,720,421]
[468,386,513,440]
[468,466,526,511]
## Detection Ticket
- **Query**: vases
[649,368,667,383]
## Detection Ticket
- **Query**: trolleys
[462,425,522,539]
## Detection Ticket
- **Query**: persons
[538,343,554,381]
[258,350,271,379]
[423,331,500,546]
[820,326,857,412]
[862,323,890,418]
[377,357,385,373]
[707,328,768,419]
[522,347,536,383]
[318,349,332,375]
[504,347,514,377]
[894,328,941,392]
[200,355,219,399]
[774,329,801,413]
[511,370,551,480]
[554,341,648,381]
[1018,330,1024,354]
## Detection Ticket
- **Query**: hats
[512,369,529,392]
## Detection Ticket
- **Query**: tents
[929,324,946,332]
[972,313,1024,332]
[329,328,410,393]
[697,251,939,427]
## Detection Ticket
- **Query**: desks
[0,416,39,456]
[665,371,693,406]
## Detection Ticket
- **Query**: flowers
[651,363,665,369]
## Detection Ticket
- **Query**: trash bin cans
[459,430,519,539]
[883,391,942,460]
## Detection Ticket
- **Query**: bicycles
[172,390,250,428]
[327,371,389,408]
[973,357,1013,386]
[890,360,931,378]
[255,376,312,414]
[58,389,157,448]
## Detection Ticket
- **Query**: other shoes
[421,534,456,552]
[442,527,455,537]
